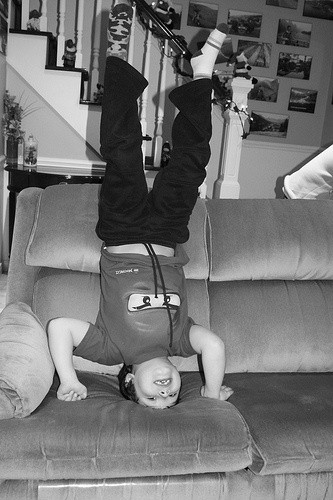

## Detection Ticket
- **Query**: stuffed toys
[27,9,43,31]
[95,83,104,102]
[234,49,258,84]
[154,0,176,31]
[160,142,172,167]
[61,39,77,68]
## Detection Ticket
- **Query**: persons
[47,4,235,409]
[285,26,293,45]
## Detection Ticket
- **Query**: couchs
[0,184,333,500]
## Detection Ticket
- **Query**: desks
[5,163,106,258]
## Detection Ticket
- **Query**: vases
[6,134,18,168]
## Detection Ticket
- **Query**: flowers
[1,87,41,145]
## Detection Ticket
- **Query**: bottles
[23,135,38,167]
